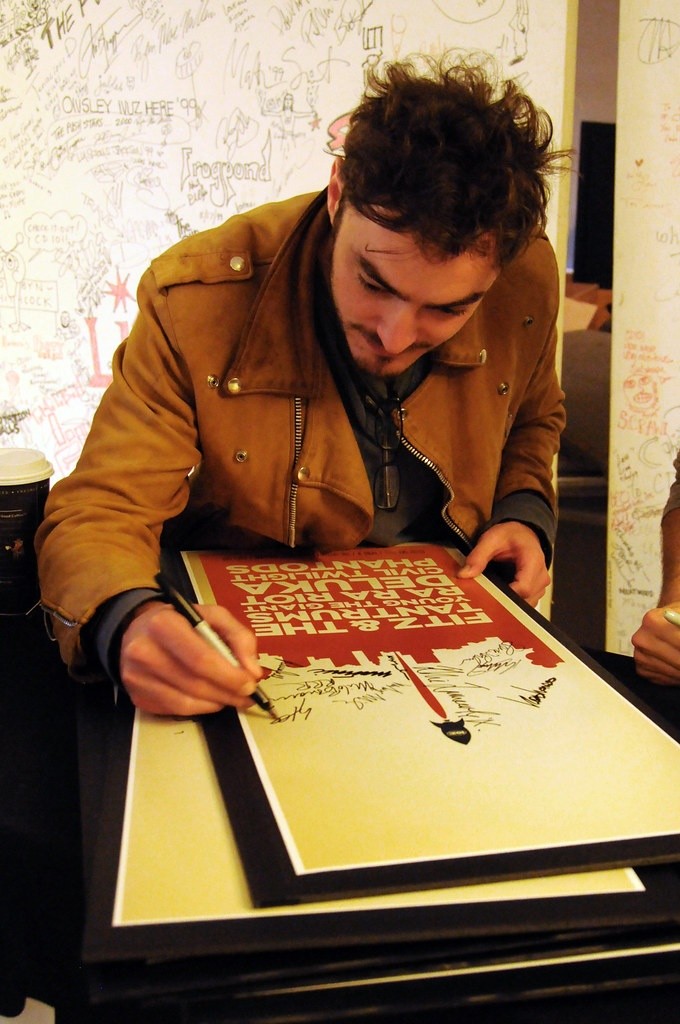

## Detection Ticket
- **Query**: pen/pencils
[148,573,285,728]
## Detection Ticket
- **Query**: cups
[0,447,54,602]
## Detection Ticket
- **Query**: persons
[36,57,567,716]
[632,449,680,684]
